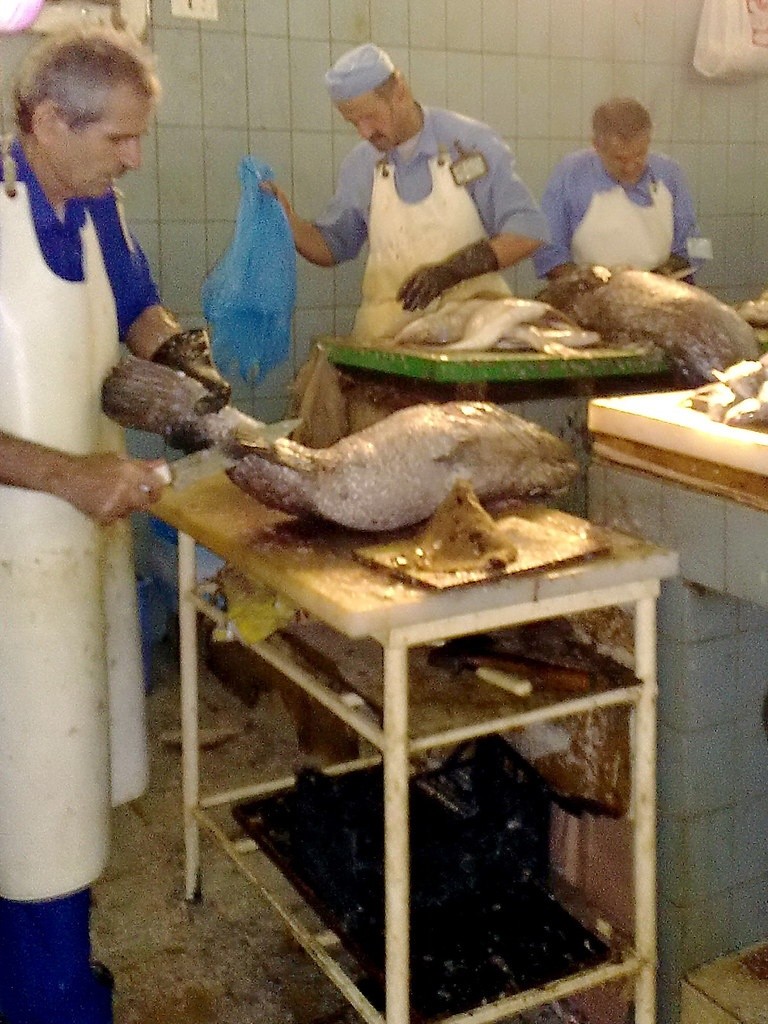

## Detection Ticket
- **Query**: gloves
[149,327,231,455]
[396,238,500,313]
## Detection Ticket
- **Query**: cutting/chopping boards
[581,380,767,480]
[137,452,681,641]
[309,328,680,385]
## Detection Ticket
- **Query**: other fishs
[101,265,768,571]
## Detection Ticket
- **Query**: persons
[532,100,706,284]
[258,39,550,334]
[0,29,229,1024]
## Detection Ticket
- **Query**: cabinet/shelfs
[144,475,680,1024]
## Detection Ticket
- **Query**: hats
[323,43,395,103]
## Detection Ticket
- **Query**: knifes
[136,416,307,499]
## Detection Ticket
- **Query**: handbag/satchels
[200,156,296,384]
